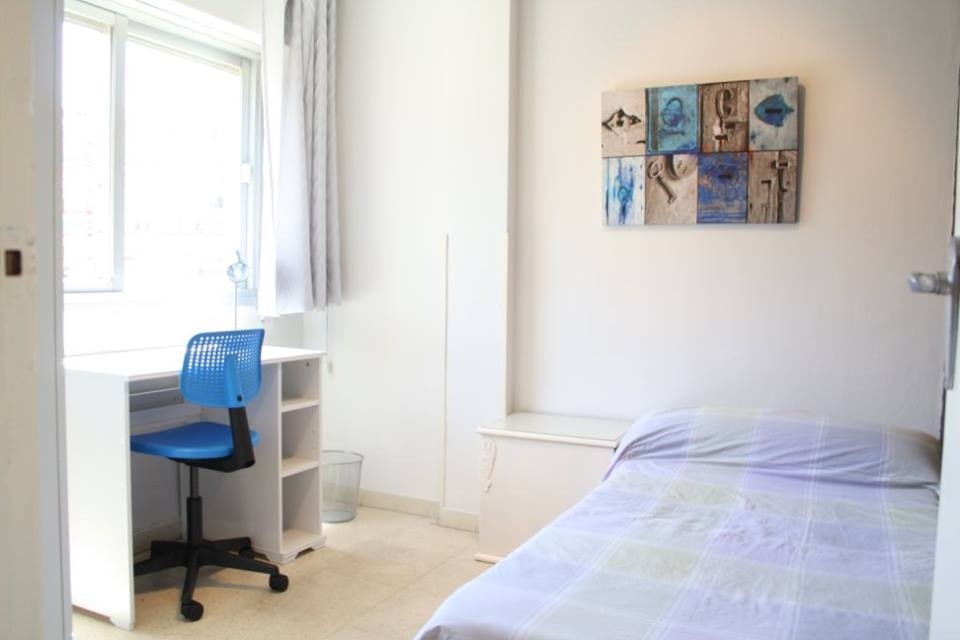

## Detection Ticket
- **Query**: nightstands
[469,413,627,564]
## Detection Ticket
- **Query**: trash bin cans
[320,450,364,523]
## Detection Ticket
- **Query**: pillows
[619,408,940,486]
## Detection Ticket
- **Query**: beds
[413,402,939,640]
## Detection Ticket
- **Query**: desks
[63,341,326,629]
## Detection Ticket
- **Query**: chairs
[131,329,291,621]
